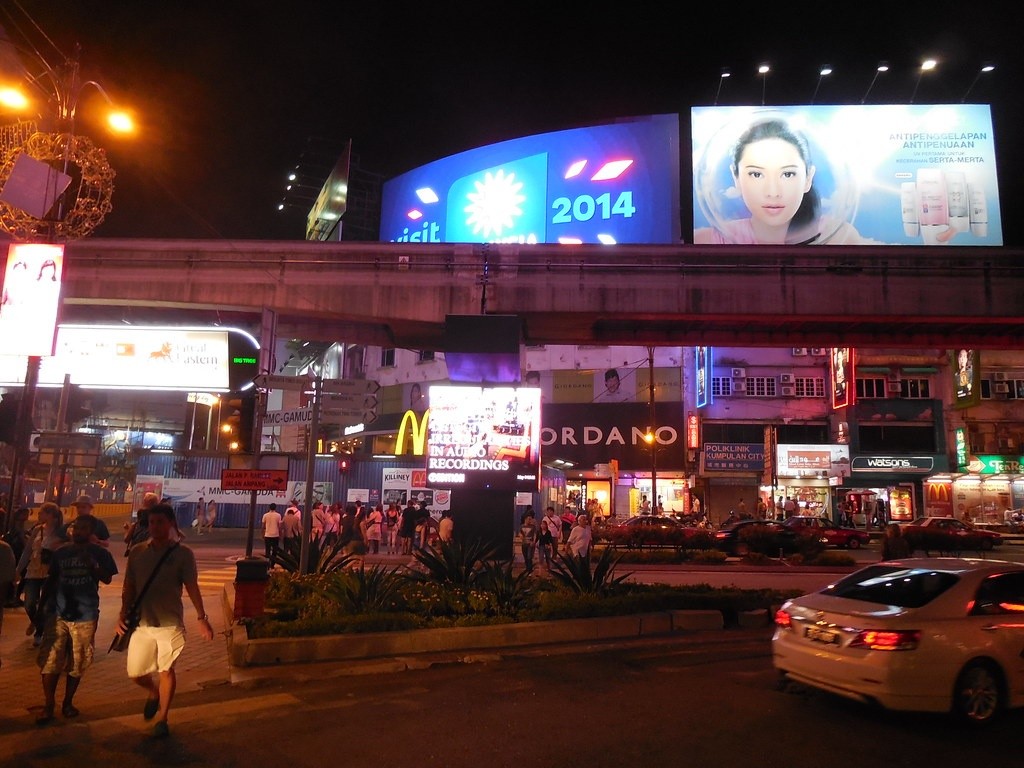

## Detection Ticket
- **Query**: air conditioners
[731,367,747,391]
[992,372,1009,393]
[779,373,795,395]
[792,347,827,356]
[886,370,902,392]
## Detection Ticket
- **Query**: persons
[960,511,976,529]
[196,496,216,536]
[1004,507,1024,535]
[783,496,822,526]
[534,520,553,572]
[559,506,576,527]
[598,369,636,402]
[691,493,702,513]
[639,495,652,515]
[694,119,863,244]
[122,492,186,559]
[836,501,859,530]
[565,515,595,559]
[736,498,746,516]
[525,371,550,403]
[261,500,455,559]
[542,506,562,559]
[585,498,621,527]
[519,505,537,578]
[756,497,775,521]
[114,504,215,738]
[775,496,785,521]
[0,495,120,725]
[0,508,16,640]
[34,514,119,725]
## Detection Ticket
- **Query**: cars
[772,558,1024,725]
[714,520,828,558]
[783,516,870,548]
[606,515,713,551]
[898,517,1002,551]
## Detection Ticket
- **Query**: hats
[291,499,298,506]
[71,496,94,507]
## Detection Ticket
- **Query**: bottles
[16,575,21,584]
[67,523,75,543]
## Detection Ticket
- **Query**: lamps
[909,58,937,104]
[810,64,833,106]
[713,66,731,104]
[961,61,996,103]
[279,136,345,212]
[860,60,890,105]
[759,61,770,107]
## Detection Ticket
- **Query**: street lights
[0,38,141,535]
[644,433,657,516]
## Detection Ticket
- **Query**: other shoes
[16,598,24,606]
[387,551,412,554]
[155,720,169,740]
[144,696,160,720]
[26,623,44,646]
[37,703,78,725]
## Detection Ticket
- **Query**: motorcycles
[672,510,751,528]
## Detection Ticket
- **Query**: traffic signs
[252,375,312,390]
[322,379,382,395]
[321,394,378,410]
[320,409,378,424]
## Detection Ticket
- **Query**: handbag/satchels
[366,519,375,529]
[108,611,137,654]
[393,514,397,523]
[124,550,130,557]
[559,532,562,539]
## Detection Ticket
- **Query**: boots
[537,566,552,577]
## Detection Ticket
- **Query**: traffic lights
[340,459,348,475]
